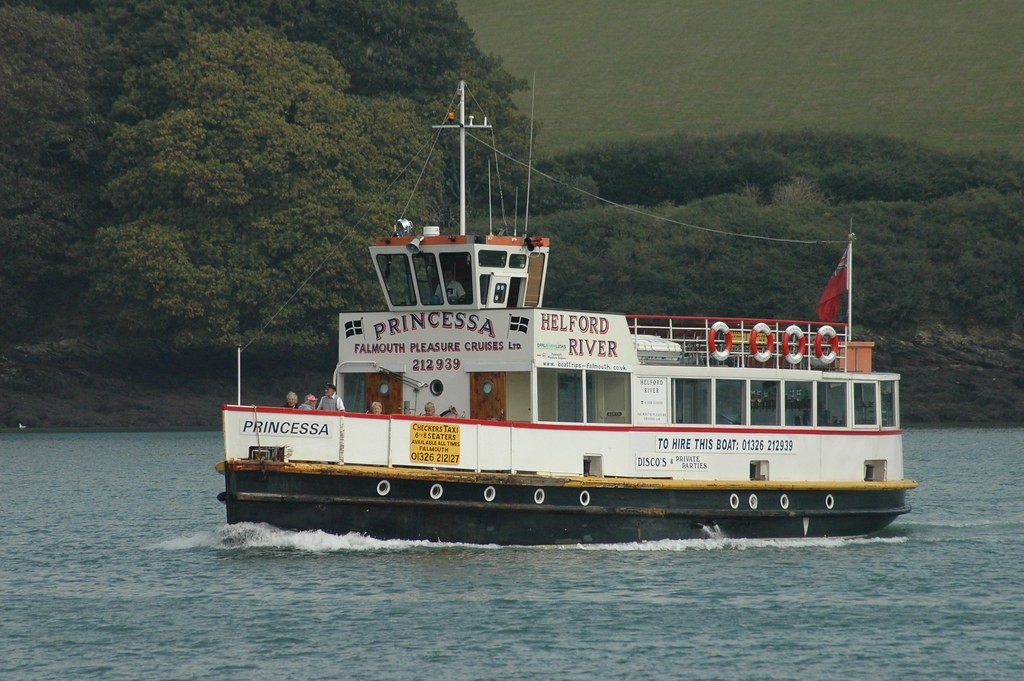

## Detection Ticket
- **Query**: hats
[306,394,318,401]
[324,383,336,391]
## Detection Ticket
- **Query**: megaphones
[406,238,420,254]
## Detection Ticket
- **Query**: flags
[816,249,848,322]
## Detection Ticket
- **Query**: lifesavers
[709,321,733,362]
[750,322,774,363]
[814,325,838,364]
[782,325,806,365]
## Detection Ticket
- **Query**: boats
[214,68,919,547]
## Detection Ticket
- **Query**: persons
[435,270,466,303]
[317,383,345,411]
[419,402,439,417]
[281,392,298,409]
[298,393,318,410]
[371,402,382,414]
